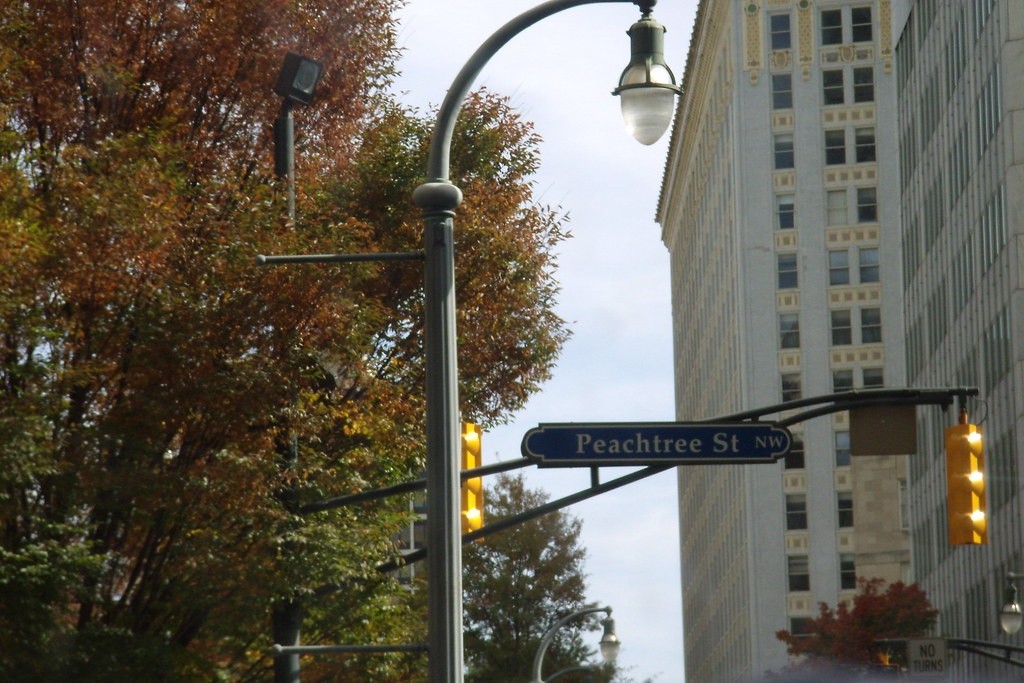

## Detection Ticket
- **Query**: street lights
[406,0,685,683]
